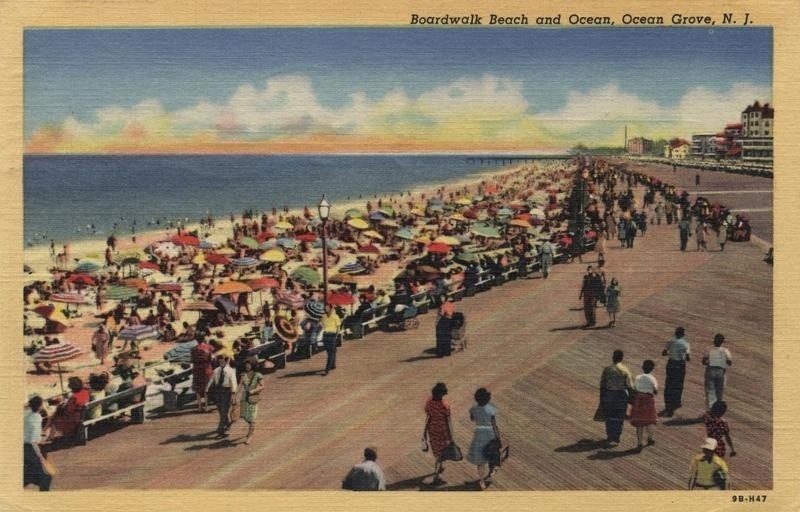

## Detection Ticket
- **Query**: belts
[694,482,720,489]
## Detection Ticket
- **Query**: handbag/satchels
[230,401,243,422]
[442,442,464,462]
[245,391,263,405]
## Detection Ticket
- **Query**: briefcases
[488,442,511,466]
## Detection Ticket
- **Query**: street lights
[319,194,330,306]
[577,167,590,261]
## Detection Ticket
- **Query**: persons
[25,160,753,491]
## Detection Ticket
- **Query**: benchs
[78,384,147,441]
[155,368,195,410]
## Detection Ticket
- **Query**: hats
[700,436,719,451]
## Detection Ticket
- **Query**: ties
[216,367,225,387]
